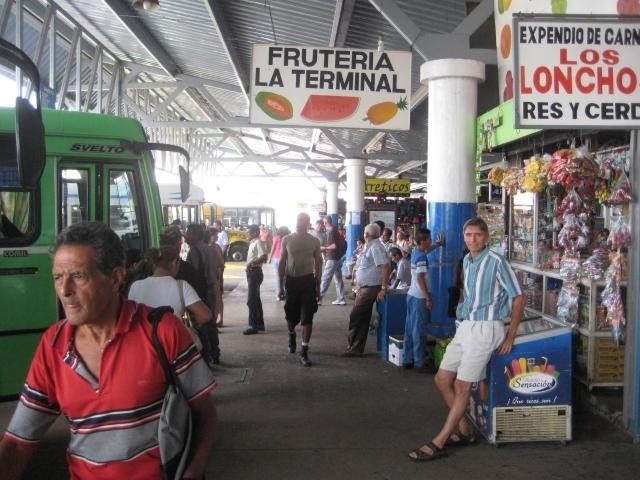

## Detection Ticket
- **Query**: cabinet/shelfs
[510,262,628,391]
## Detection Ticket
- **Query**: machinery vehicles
[203,200,251,261]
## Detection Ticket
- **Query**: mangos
[255,91,293,121]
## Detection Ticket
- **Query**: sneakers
[243,327,265,334]
[404,362,436,372]
[332,298,346,304]
[341,345,362,356]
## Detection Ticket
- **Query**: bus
[222,205,276,231]
[1,35,51,190]
[0,103,201,388]
[60,177,206,259]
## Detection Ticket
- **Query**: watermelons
[299,93,359,123]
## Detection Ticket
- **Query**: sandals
[408,443,449,460]
[445,429,478,446]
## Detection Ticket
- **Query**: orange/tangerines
[499,25,513,59]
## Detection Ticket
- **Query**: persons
[127,213,446,373]
[0,222,218,480]
[408,219,524,462]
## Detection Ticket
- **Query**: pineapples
[367,98,408,125]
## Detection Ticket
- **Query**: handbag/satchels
[184,310,204,352]
[157,385,199,479]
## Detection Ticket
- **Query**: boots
[298,345,312,366]
[289,333,296,353]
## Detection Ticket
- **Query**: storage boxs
[388,335,405,367]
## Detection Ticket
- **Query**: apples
[502,71,514,101]
